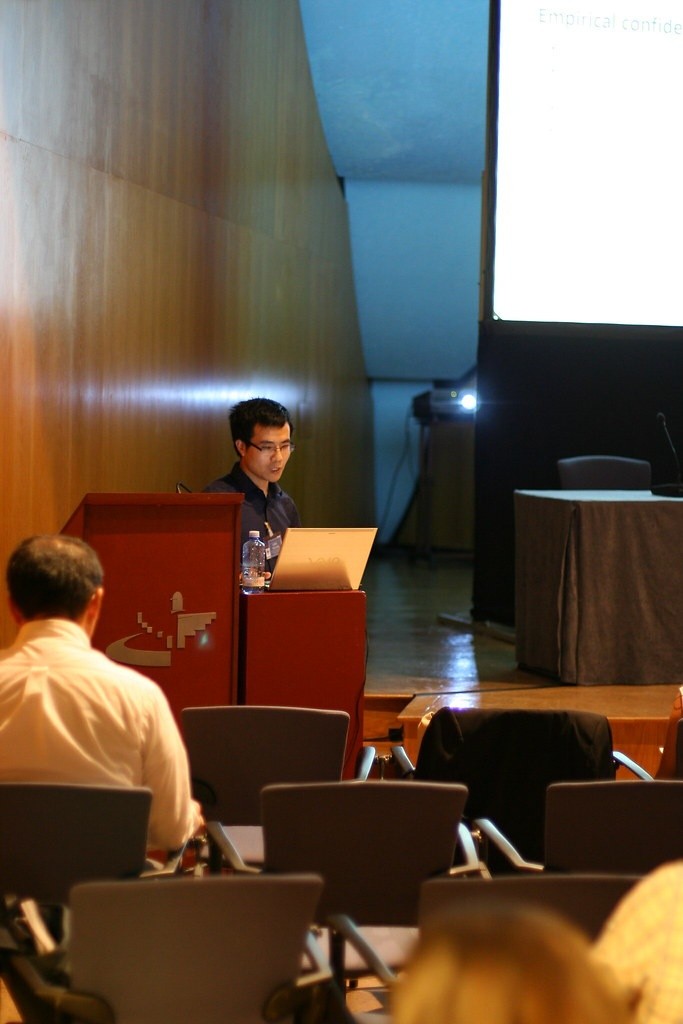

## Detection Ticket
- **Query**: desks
[508,489,683,683]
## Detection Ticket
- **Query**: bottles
[241,531,266,595]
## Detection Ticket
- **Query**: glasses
[244,440,296,456]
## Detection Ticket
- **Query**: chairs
[204,779,480,930]
[2,780,154,909]
[1,871,324,1024]
[385,706,655,873]
[328,872,650,1024]
[180,704,377,875]
[559,456,653,489]
[472,780,683,876]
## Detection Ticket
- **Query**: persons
[197,398,303,590]
[585,862,682,1024]
[382,901,638,1024]
[0,533,205,876]
